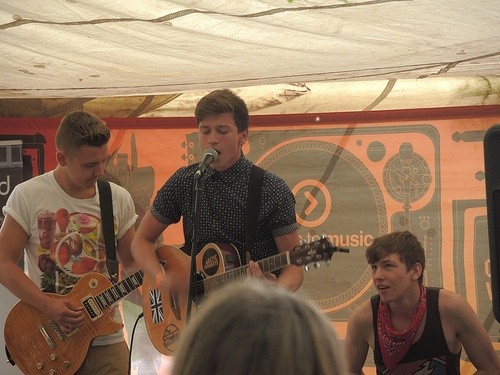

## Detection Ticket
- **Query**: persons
[342,230,500,375]
[130,90,305,295]
[0,109,139,375]
[169,279,347,375]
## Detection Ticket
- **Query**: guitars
[3,261,162,374]
[143,237,351,355]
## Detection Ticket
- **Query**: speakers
[483,123,500,323]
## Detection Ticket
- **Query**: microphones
[194,148,218,179]
